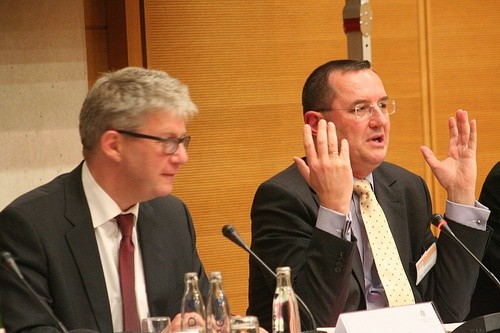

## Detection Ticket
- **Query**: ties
[114,213,141,333]
[353,179,415,307]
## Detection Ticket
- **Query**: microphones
[222,225,327,333]
[0,252,68,333]
[430,213,500,333]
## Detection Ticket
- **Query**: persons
[0,67,213,333]
[246,59,500,333]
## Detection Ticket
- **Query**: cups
[142,316,170,333]
[230,316,259,333]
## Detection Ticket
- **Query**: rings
[329,151,338,154]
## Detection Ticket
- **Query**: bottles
[271,267,301,333]
[181,272,206,333]
[206,272,230,333]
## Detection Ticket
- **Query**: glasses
[115,130,191,155]
[315,99,395,118]
